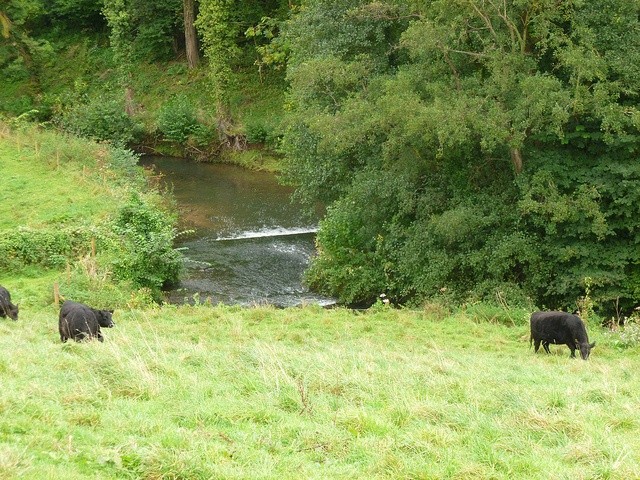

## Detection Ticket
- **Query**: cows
[86,307,116,329]
[58,299,104,344]
[529,311,596,361]
[0,284,20,321]
